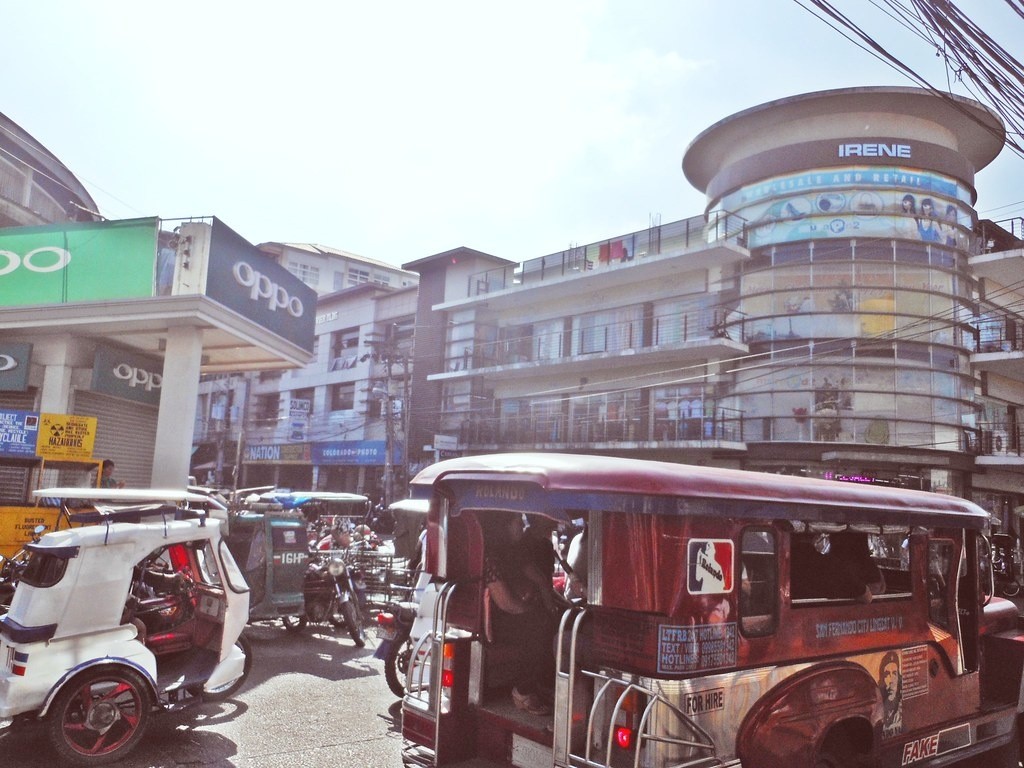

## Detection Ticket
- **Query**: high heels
[512,689,552,715]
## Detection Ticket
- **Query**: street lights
[369,386,395,514]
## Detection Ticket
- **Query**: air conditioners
[984,430,1007,454]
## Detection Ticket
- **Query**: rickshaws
[0,477,432,768]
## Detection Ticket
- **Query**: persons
[478,509,582,715]
[782,524,891,606]
[93,459,126,489]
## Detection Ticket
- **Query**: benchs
[483,589,528,663]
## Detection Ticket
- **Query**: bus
[393,450,1024,768]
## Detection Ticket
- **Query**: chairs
[132,609,191,657]
